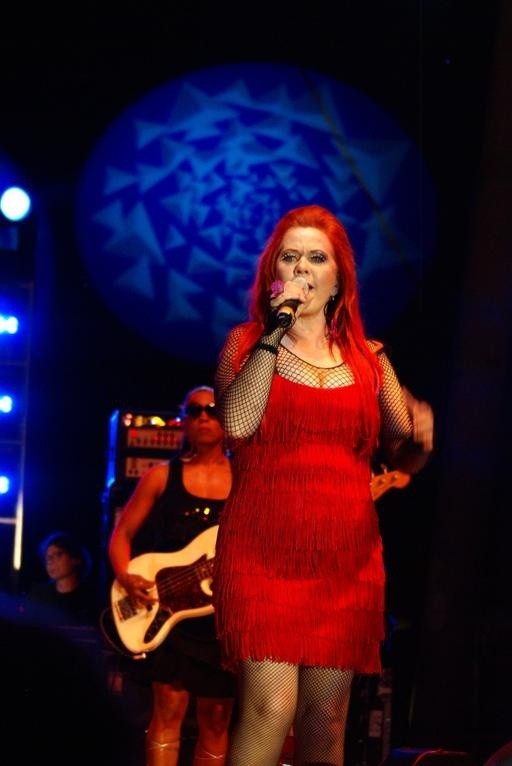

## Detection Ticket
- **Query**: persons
[37,528,92,594]
[207,200,438,766]
[105,380,250,765]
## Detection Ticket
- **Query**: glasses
[184,402,217,417]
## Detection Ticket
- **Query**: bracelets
[254,341,282,359]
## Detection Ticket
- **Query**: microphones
[275,277,309,326]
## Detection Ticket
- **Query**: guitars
[111,464,410,654]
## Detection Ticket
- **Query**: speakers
[100,449,176,622]
